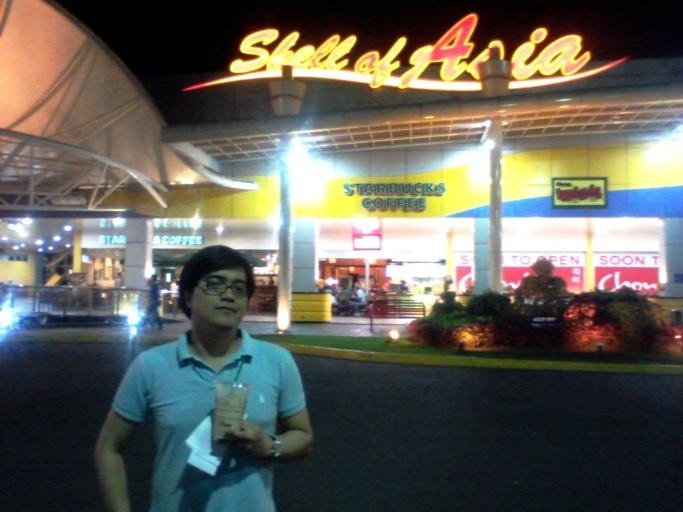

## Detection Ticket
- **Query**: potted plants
[562,290,653,361]
[407,290,527,353]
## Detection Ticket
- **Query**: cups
[211,378,248,441]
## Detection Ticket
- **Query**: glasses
[199,279,249,296]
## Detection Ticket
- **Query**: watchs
[268,435,282,460]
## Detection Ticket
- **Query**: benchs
[368,302,425,331]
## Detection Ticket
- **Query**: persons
[94,245,314,512]
[139,274,163,331]
[400,280,409,291]
[330,282,368,317]
